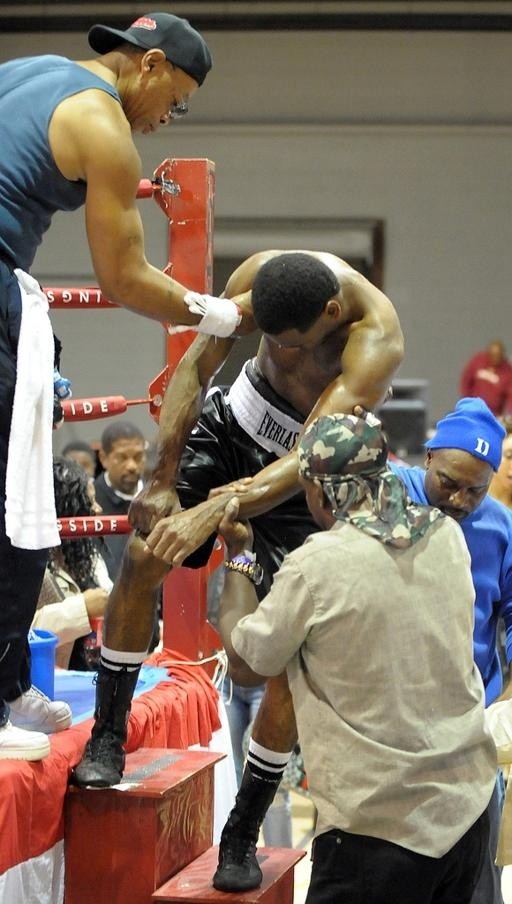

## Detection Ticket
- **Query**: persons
[52,339,512,715]
[1,8,213,763]
[217,407,505,904]
[384,396,512,904]
[67,252,407,893]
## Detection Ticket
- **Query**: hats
[297,411,389,484]
[424,397,508,474]
[87,11,212,88]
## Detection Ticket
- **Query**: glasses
[163,57,189,121]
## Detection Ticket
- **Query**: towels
[3,267,63,552]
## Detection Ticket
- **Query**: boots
[72,656,143,788]
[213,759,284,891]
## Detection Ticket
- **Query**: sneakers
[5,683,72,733]
[1,720,51,763]
